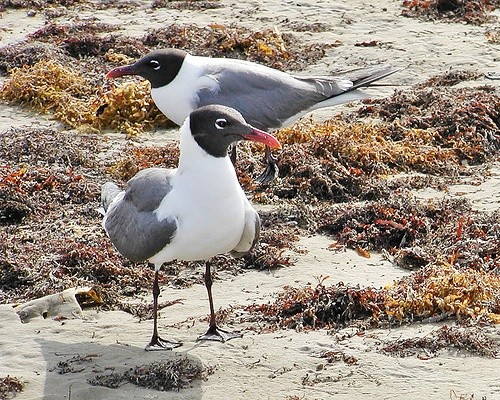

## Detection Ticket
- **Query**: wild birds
[93,103,281,351]
[106,48,402,182]
